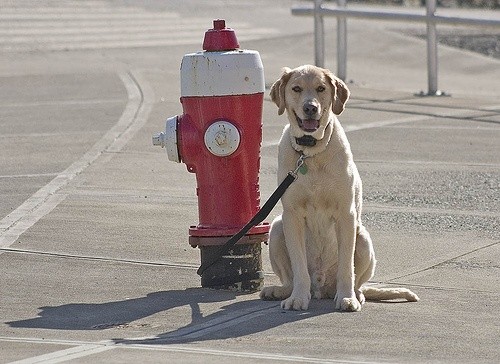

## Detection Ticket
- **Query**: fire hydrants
[151,20,270,292]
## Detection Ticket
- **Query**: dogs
[258,64,420,312]
